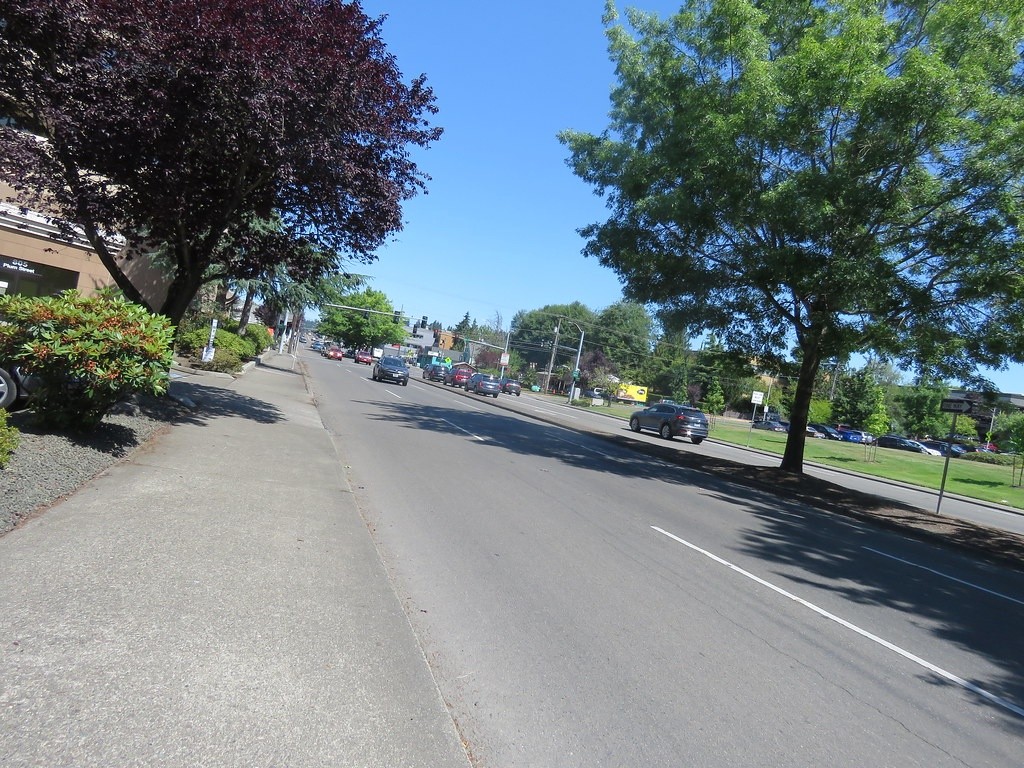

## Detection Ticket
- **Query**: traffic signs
[939,398,973,414]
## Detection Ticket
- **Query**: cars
[443,368,473,388]
[423,363,450,382]
[355,351,373,366]
[752,418,1016,458]
[372,354,410,386]
[299,332,355,361]
[464,372,502,399]
[0,312,99,415]
[585,384,695,408]
[500,377,522,396]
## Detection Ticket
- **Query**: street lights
[567,322,585,405]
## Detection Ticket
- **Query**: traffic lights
[434,328,439,338]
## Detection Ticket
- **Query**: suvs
[629,403,710,445]
